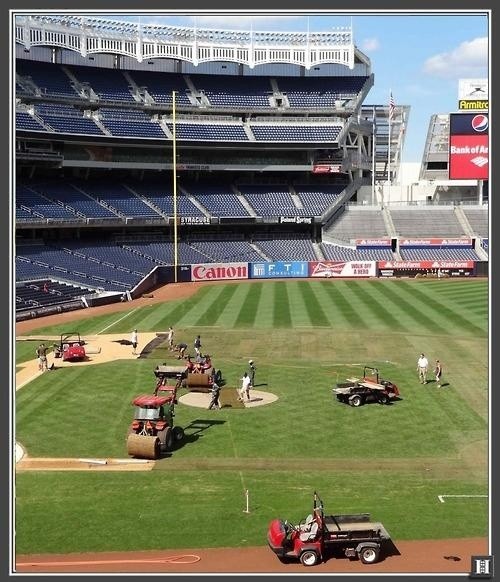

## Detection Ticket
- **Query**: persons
[174,343,187,360]
[239,372,252,403]
[129,329,139,355]
[209,381,222,410]
[249,359,258,388]
[416,353,429,384]
[167,326,175,352]
[433,359,442,390]
[44,283,48,294]
[36,345,41,370]
[192,335,204,359]
[35,344,53,371]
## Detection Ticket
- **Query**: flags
[389,95,396,120]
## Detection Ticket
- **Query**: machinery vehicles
[126,363,184,456]
[180,354,223,391]
[268,491,391,565]
[334,365,399,405]
[53,332,86,360]
